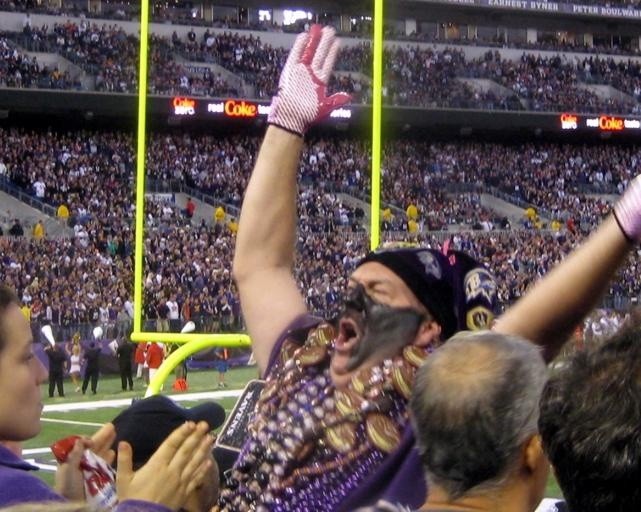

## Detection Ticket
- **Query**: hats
[111,395,226,471]
[355,249,458,340]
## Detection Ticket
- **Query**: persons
[85,394,225,512]
[0,278,217,512]
[533,315,640,512]
[200,21,641,511]
[408,330,548,512]
[1,119,641,340]
[43,335,229,390]
[1,0,641,117]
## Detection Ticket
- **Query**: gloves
[265,23,353,138]
